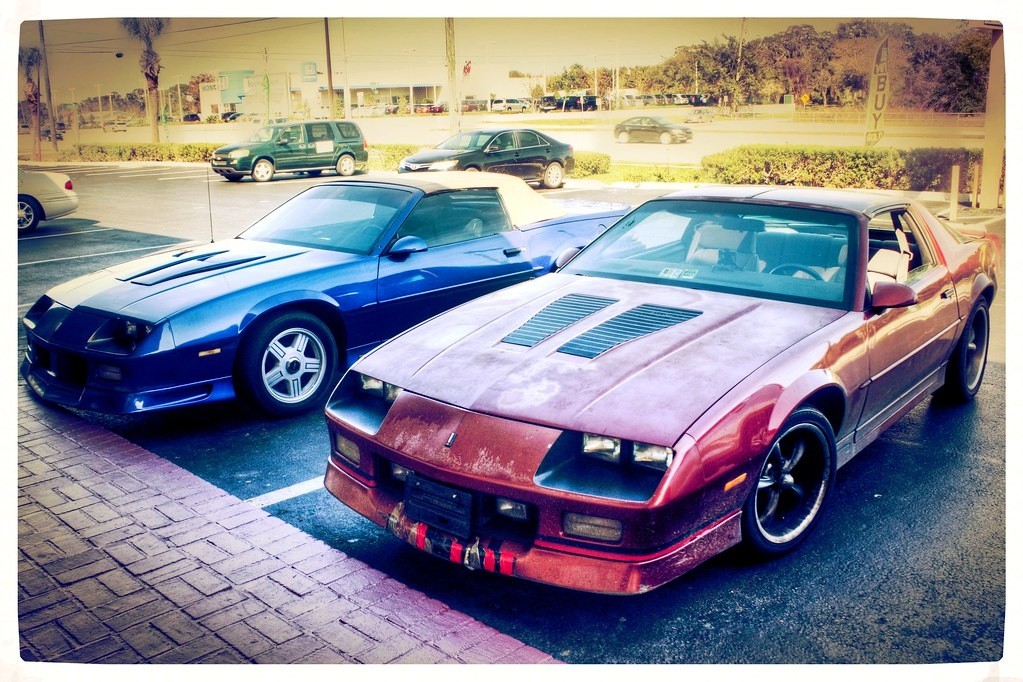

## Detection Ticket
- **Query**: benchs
[756,231,923,282]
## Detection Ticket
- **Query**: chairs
[281,131,290,144]
[835,245,900,295]
[689,217,765,273]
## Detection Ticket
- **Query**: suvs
[209,120,371,182]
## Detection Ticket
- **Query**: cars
[18,165,81,235]
[612,117,697,146]
[182,113,202,123]
[106,118,128,134]
[397,130,578,189]
[220,110,246,122]
[358,92,720,116]
[19,118,70,144]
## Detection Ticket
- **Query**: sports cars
[20,173,632,420]
[323,185,998,597]
